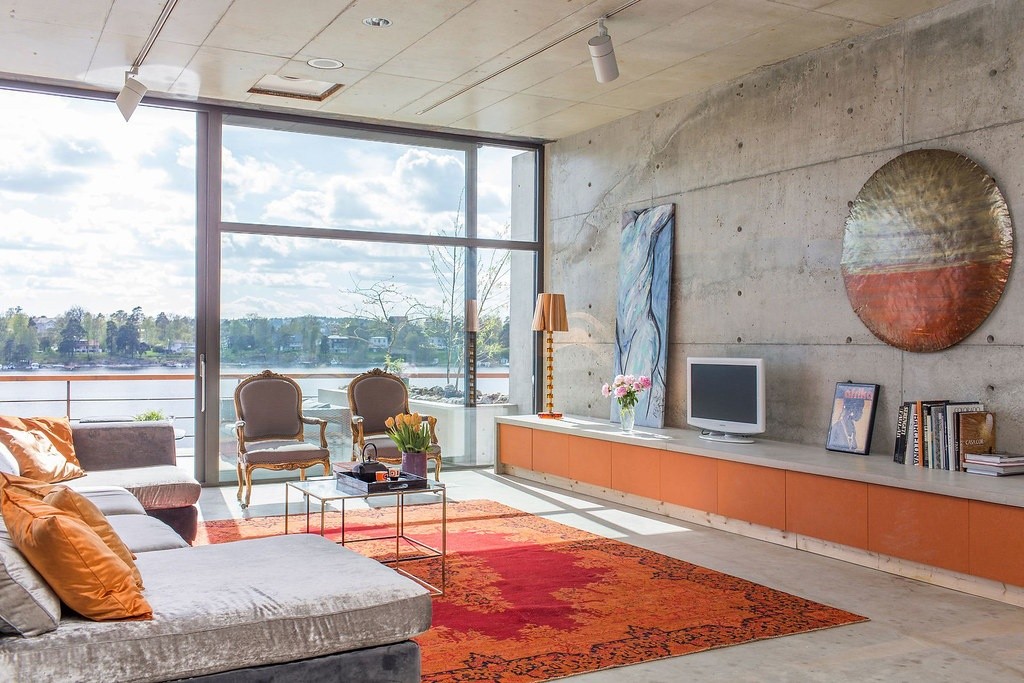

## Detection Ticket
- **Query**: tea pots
[352,443,387,483]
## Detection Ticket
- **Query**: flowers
[602,374,652,416]
[384,413,436,454]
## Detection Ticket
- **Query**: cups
[376,471,388,483]
[389,468,400,477]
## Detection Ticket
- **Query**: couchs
[0,421,432,683]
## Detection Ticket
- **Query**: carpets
[192,499,871,683]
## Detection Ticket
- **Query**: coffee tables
[285,478,447,597]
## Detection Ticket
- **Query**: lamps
[462,299,478,408]
[530,293,569,419]
[116,64,148,123]
[588,13,620,84]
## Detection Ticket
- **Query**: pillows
[0,415,153,622]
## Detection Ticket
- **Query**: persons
[829,399,864,449]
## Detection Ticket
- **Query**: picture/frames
[825,382,880,456]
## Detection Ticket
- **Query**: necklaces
[841,420,853,450]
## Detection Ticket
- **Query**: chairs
[234,369,330,508]
[347,368,441,495]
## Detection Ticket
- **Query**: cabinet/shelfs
[494,413,1024,610]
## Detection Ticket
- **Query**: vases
[401,451,427,479]
[619,398,635,431]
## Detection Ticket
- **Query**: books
[893,401,1024,477]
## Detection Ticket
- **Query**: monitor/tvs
[685,357,766,444]
